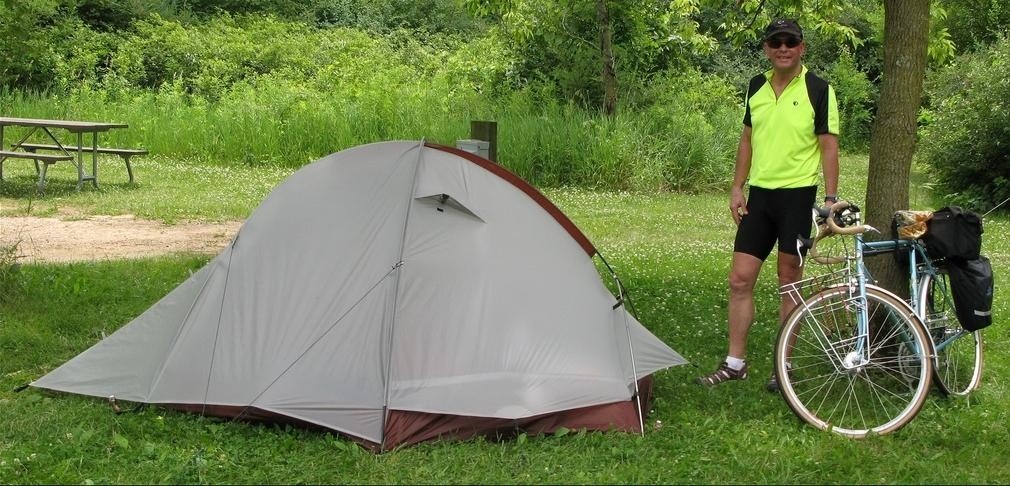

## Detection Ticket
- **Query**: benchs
[0,150,73,185]
[11,142,150,183]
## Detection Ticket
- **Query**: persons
[698,19,840,390]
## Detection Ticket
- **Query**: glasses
[763,38,802,49]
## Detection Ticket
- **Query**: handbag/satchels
[891,206,994,333]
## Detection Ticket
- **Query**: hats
[763,18,802,38]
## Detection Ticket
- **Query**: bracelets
[825,196,837,203]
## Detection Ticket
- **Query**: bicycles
[768,195,997,435]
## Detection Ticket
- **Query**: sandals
[766,362,792,394]
[695,363,747,385]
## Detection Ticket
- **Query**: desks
[0,116,129,191]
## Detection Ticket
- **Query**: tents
[28,140,698,453]
